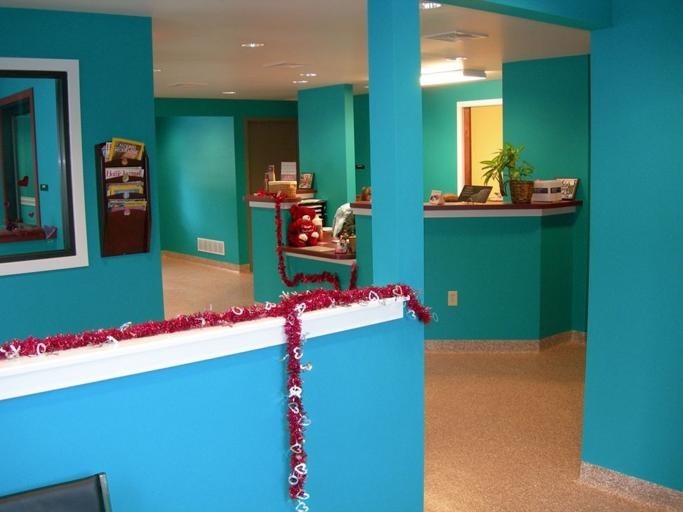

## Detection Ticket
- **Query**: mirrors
[0,59,91,279]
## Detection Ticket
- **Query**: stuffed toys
[285,205,319,247]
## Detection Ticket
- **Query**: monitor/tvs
[457,185,493,203]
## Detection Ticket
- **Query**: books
[99,138,147,211]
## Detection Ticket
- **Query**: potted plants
[479,142,535,205]
